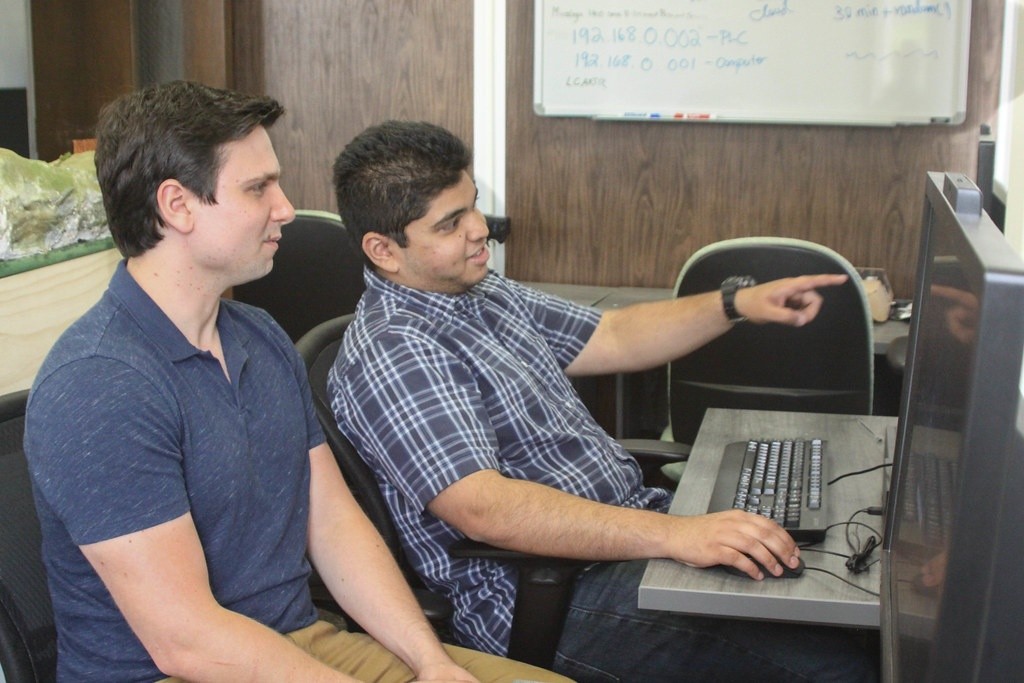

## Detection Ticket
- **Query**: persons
[21,74,574,683]
[325,125,882,678]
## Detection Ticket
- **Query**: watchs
[720,273,758,326]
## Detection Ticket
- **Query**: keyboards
[707,440,831,543]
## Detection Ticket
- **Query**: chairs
[240,209,366,346]
[674,236,876,444]
[0,389,453,683]
[294,312,692,671]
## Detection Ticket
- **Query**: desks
[637,408,899,628]
[899,426,961,644]
[514,279,910,438]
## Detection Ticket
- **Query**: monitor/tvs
[881,164,1024,683]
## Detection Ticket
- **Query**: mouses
[722,552,806,579]
